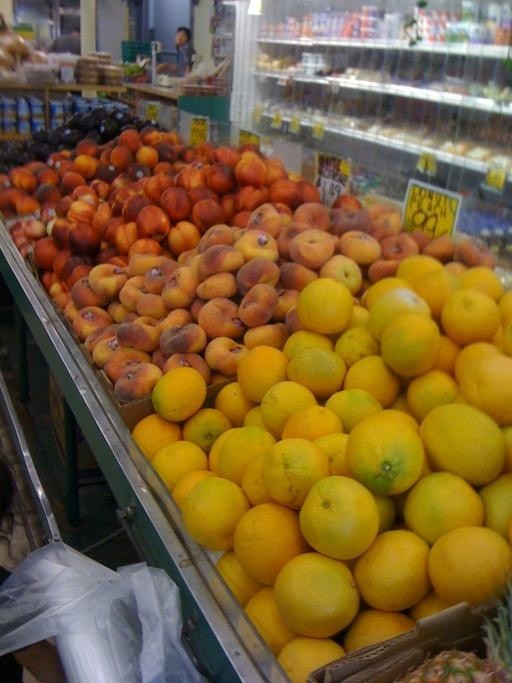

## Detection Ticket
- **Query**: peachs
[0,127,497,402]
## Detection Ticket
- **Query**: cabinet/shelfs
[0,82,128,143]
[252,0,512,240]
[0,212,292,683]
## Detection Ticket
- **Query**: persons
[44,25,81,55]
[155,26,194,77]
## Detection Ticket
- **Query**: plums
[0,108,167,174]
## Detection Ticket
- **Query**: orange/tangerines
[130,256,512,683]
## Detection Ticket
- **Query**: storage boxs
[306,598,509,683]
